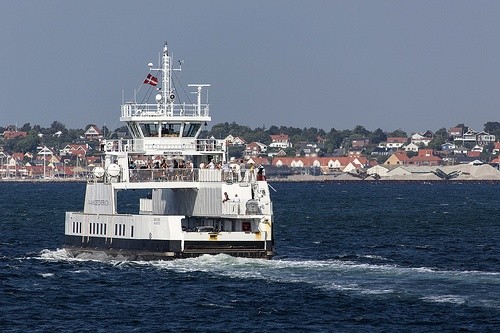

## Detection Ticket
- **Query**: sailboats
[0,146,104,182]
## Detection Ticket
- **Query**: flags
[143,73,157,86]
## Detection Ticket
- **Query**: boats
[64,40,276,261]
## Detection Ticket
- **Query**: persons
[239,159,266,180]
[162,127,177,134]
[129,157,169,182]
[223,192,240,214]
[200,158,221,169]
[173,159,192,180]
